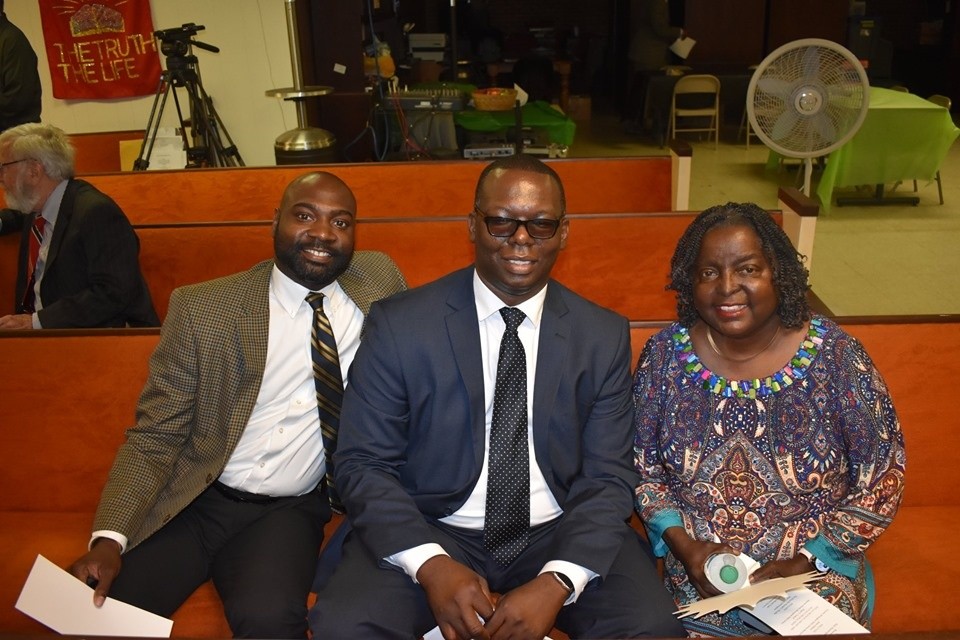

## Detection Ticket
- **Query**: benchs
[0,312,960,639]
[1,188,839,326]
[0,141,694,227]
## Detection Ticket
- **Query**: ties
[481,306,530,571]
[304,291,344,516]
[19,212,47,316]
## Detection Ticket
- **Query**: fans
[744,38,870,201]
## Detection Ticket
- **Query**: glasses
[474,205,563,240]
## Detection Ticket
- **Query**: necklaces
[706,321,782,362]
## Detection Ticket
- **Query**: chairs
[736,74,789,148]
[663,74,720,147]
[888,84,953,204]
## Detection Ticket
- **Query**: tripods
[132,53,244,168]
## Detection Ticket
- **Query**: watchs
[553,572,577,593]
[797,546,833,578]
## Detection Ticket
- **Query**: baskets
[470,87,517,111]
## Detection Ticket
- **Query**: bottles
[703,552,747,592]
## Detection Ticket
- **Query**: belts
[212,479,280,506]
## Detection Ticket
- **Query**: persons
[307,156,687,640]
[63,170,411,640]
[628,1,694,130]
[0,123,161,331]
[633,203,907,640]
[0,1,42,136]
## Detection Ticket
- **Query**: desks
[765,85,960,215]
[487,56,577,112]
[628,65,751,139]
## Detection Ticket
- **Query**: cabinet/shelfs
[294,0,420,164]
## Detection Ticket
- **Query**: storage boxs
[407,34,450,63]
[568,93,592,121]
[362,42,401,79]
